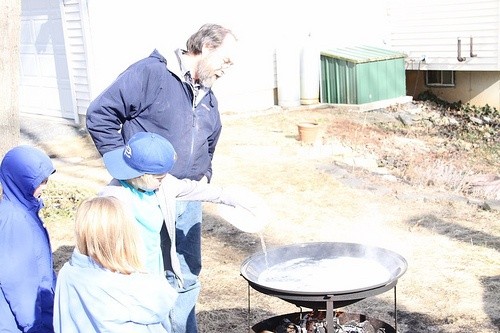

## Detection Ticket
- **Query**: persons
[85,22,240,331]
[102,131,237,332]
[0,146,58,333]
[53,195,179,331]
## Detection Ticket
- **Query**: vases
[298,122,319,141]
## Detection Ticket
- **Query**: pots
[239,240,407,312]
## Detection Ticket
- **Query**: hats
[103,132,175,180]
